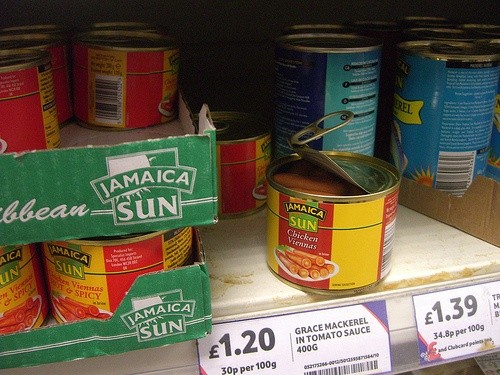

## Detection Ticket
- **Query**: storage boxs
[0,90,219,249]
[0,226,213,367]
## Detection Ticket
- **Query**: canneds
[0,15,500,334]
